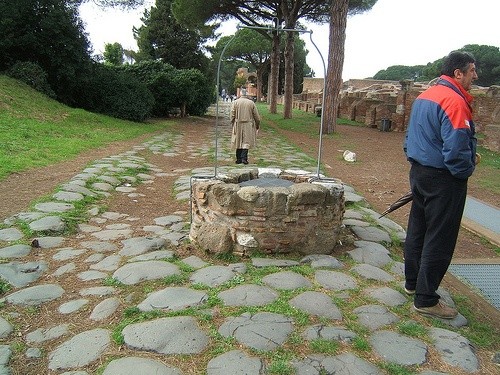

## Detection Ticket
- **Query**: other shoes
[236,160,247,164]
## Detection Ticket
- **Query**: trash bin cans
[381,118,392,131]
[316,107,322,117]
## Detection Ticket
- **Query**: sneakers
[404,287,458,319]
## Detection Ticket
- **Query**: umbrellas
[378,152,482,220]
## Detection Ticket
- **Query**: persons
[220,92,258,105]
[230,89,261,165]
[404,51,479,319]
